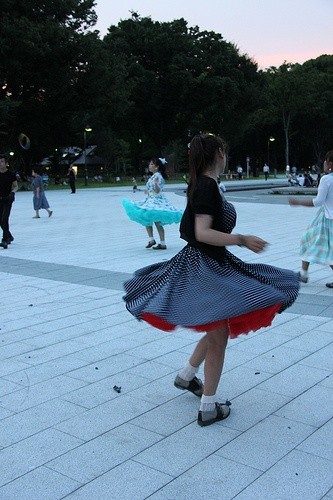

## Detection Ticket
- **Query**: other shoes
[0,237,14,249]
[326,282,333,288]
[296,271,308,284]
[153,243,167,249]
[198,402,231,427]
[146,239,156,248]
[49,211,53,217]
[174,373,203,397]
[32,216,40,218]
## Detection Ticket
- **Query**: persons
[143,173,151,184]
[286,164,328,187]
[55,178,64,184]
[264,163,269,181]
[218,181,226,191]
[0,156,18,248]
[289,155,333,288]
[132,176,136,193]
[68,166,76,193]
[238,165,243,180]
[94,175,103,182]
[32,169,53,218]
[122,133,300,426]
[123,158,184,248]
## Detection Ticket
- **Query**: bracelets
[238,234,244,247]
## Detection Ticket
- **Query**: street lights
[132,137,142,181]
[267,136,275,165]
[83,127,92,187]
